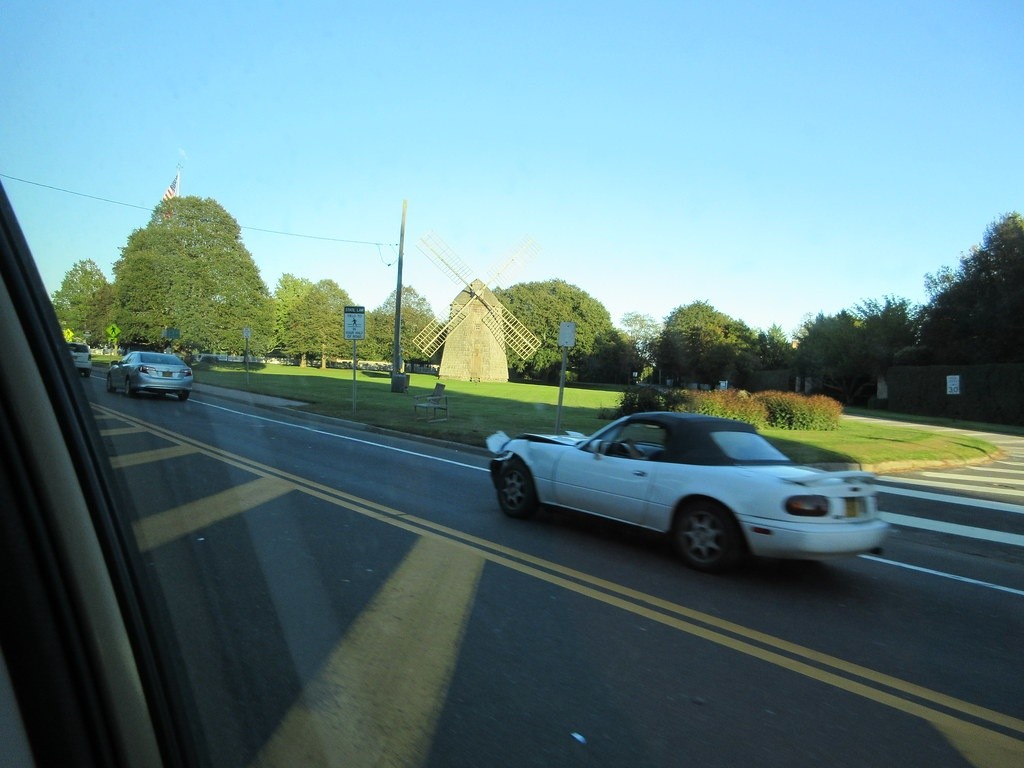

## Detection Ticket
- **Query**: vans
[67,343,93,377]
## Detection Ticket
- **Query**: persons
[625,428,672,460]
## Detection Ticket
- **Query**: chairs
[414,383,450,424]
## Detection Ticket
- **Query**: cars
[489,414,889,577]
[106,351,194,402]
